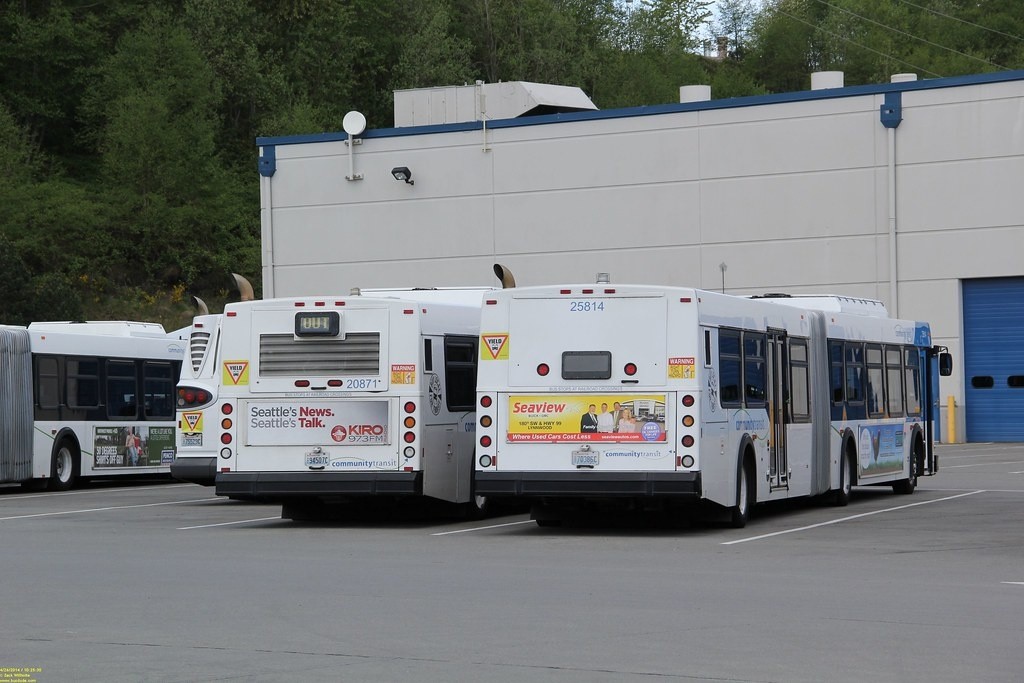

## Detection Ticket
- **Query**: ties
[592,415,596,423]
[615,412,617,424]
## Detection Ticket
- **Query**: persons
[618,410,635,433]
[125,426,138,466]
[597,403,615,433]
[609,402,636,433]
[580,404,598,432]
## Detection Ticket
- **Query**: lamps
[392,167,414,186]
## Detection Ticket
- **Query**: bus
[0,320,191,494]
[169,314,219,488]
[478,262,953,530]
[216,285,502,524]
